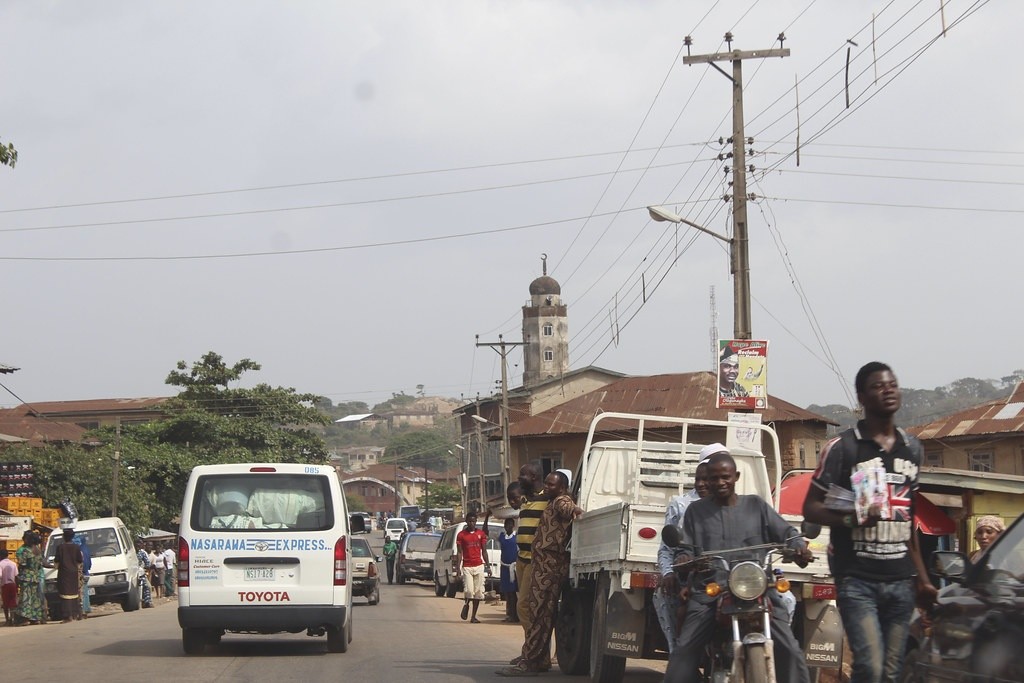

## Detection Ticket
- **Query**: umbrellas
[913,491,956,535]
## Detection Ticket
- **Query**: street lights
[396,474,416,505]
[448,449,465,514]
[471,415,511,507]
[113,465,136,518]
[647,207,752,417]
[398,466,424,495]
[455,444,485,512]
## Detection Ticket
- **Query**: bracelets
[844,514,852,529]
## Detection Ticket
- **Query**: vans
[401,505,421,527]
[395,531,442,585]
[42,515,142,614]
[348,511,372,533]
[384,518,409,544]
[174,463,363,651]
[433,521,507,598]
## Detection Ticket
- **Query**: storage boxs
[0,496,63,594]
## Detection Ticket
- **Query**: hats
[557,468,572,488]
[695,459,709,468]
[698,443,730,462]
[719,346,739,366]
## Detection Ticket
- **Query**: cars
[350,537,382,603]
[904,511,1024,683]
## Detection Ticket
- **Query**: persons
[969,515,1008,570]
[804,361,938,682]
[134,538,177,608]
[653,443,810,683]
[0,528,92,625]
[496,462,584,677]
[719,346,748,397]
[498,517,519,622]
[378,508,445,585]
[457,512,492,623]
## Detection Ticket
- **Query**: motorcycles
[662,522,820,683]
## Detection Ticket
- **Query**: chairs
[215,502,246,517]
[354,545,366,557]
[295,510,330,529]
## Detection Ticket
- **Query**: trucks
[555,412,845,682]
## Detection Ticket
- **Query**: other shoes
[510,655,550,672]
[502,617,520,624]
[471,618,481,623]
[495,665,538,677]
[460,604,469,620]
[3,613,90,626]
